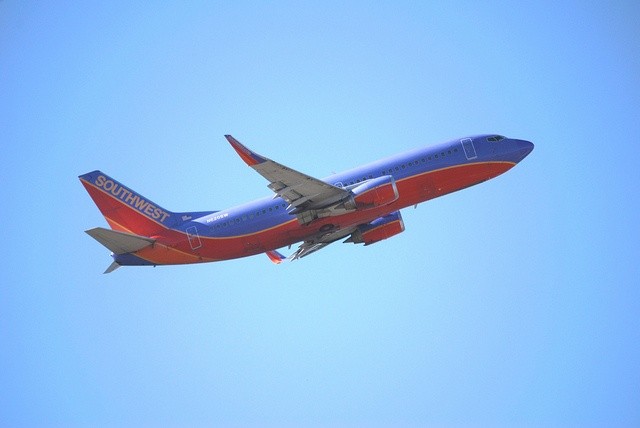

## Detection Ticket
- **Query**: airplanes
[78,134,535,275]
